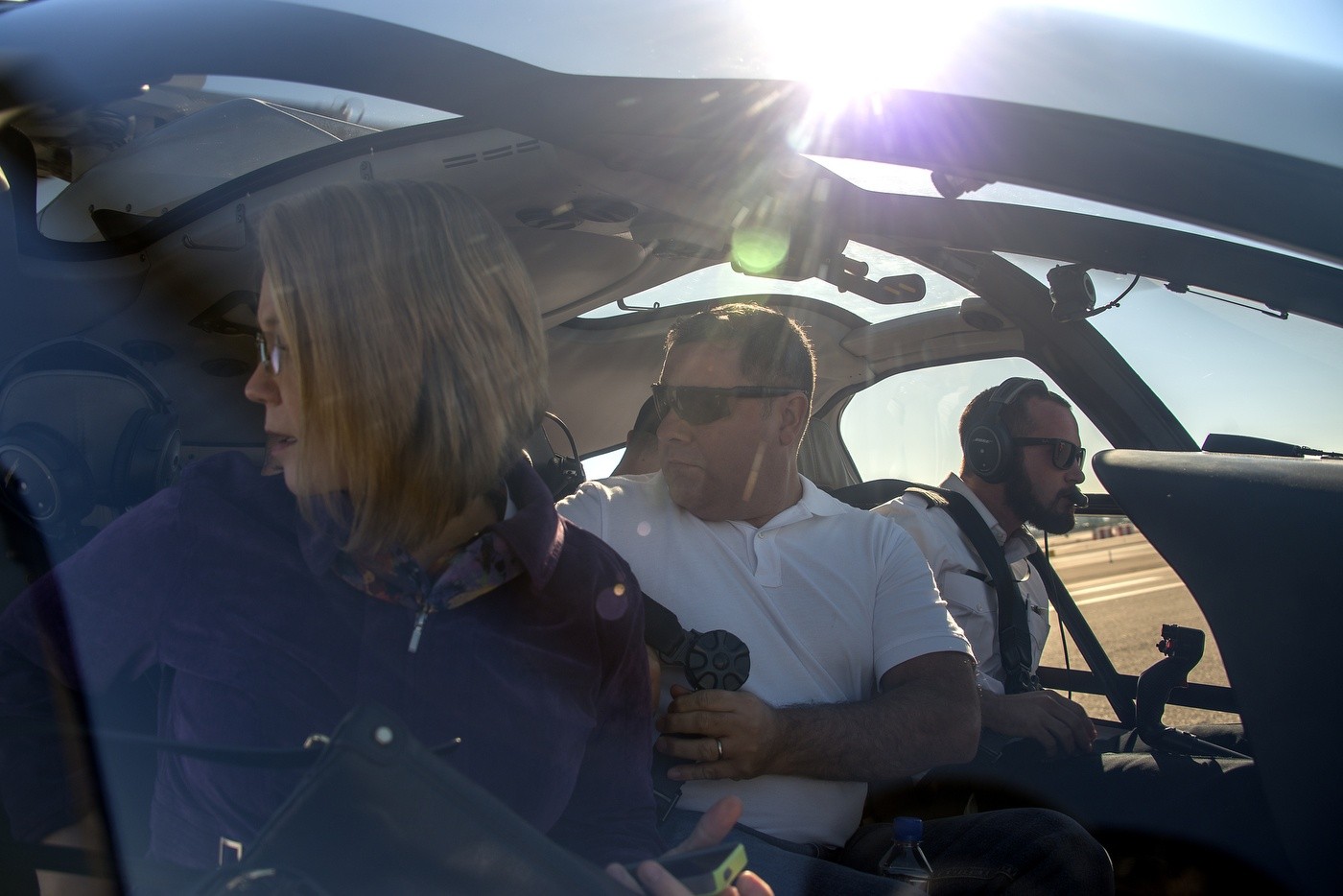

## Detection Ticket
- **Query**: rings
[714,738,724,762]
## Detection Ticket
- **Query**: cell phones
[617,843,750,895]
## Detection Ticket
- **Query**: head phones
[519,409,587,504]
[963,375,1050,486]
[1,340,181,534]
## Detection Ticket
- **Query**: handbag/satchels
[193,704,644,896]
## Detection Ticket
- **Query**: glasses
[1012,437,1086,471]
[252,331,292,376]
[650,381,809,425]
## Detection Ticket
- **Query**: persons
[860,376,1171,895]
[0,173,775,896]
[609,395,663,478]
[552,301,1119,896]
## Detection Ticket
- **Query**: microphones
[1073,489,1089,509]
